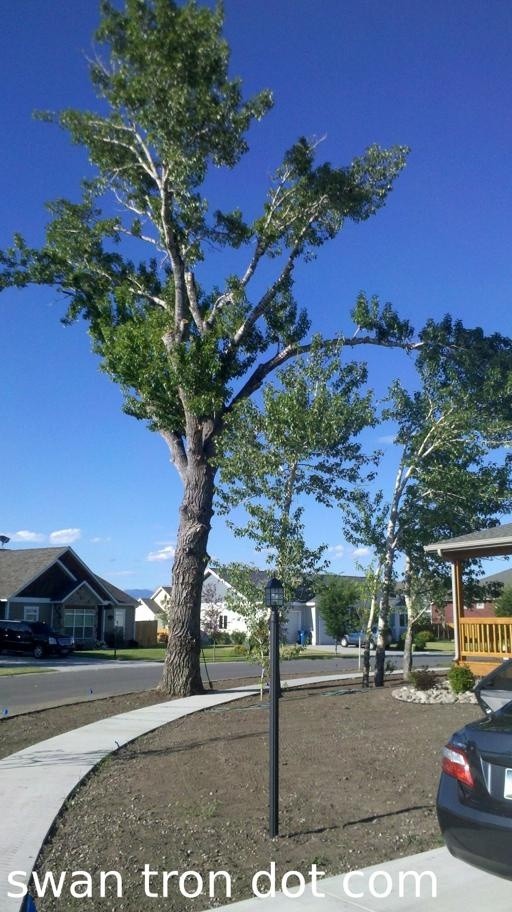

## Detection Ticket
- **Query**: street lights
[262,569,286,842]
[113,619,119,658]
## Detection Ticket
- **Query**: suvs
[1,619,75,660]
[339,623,392,649]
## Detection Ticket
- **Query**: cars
[437,656,512,881]
